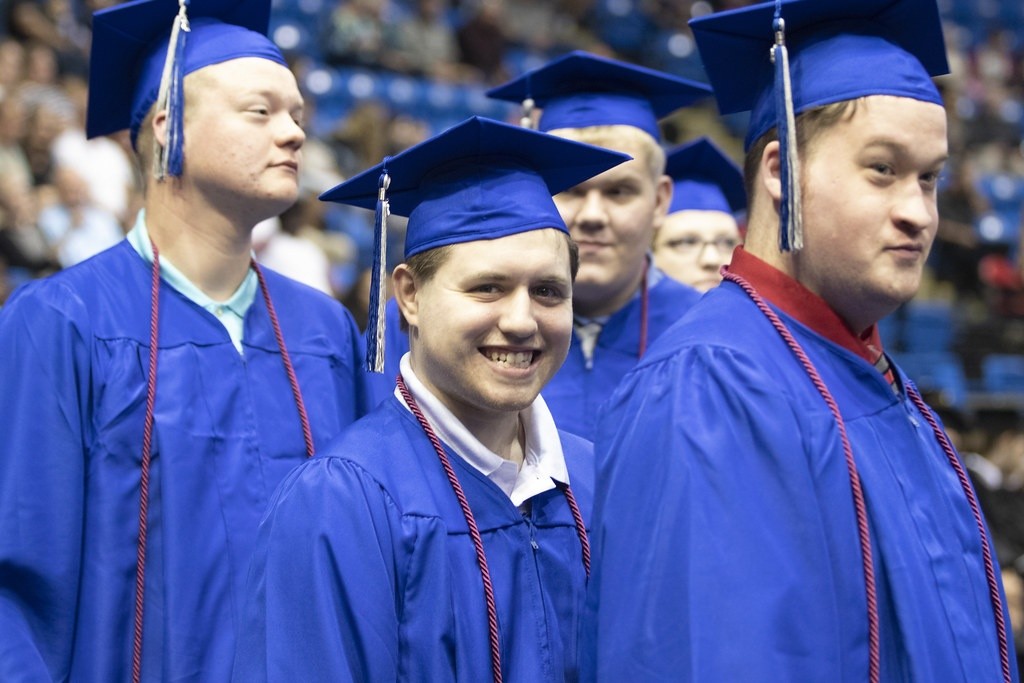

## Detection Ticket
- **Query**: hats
[687,0,951,252]
[318,115,635,374]
[485,51,715,135]
[663,137,751,218]
[86,0,291,181]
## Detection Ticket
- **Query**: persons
[230,116,632,683]
[0,0,1024,683]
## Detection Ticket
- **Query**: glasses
[653,231,744,266]
